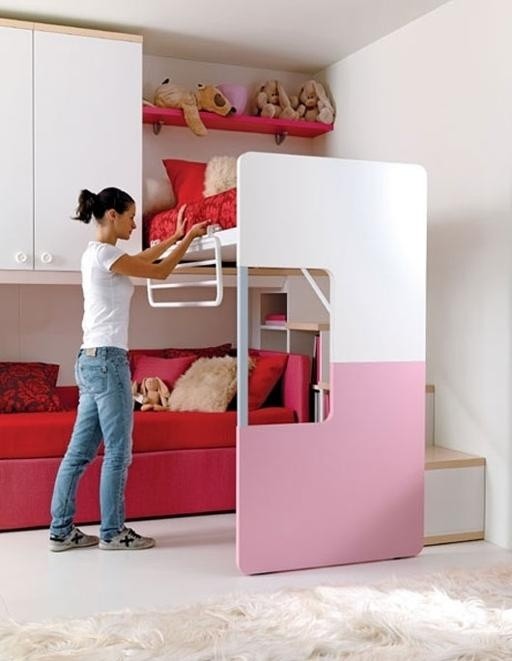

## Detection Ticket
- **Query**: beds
[146,152,429,576]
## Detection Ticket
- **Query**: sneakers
[49,527,98,552]
[99,528,155,550]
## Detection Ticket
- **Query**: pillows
[168,357,256,414]
[1,360,65,413]
[132,354,196,393]
[161,158,207,206]
[128,343,234,360]
[247,353,289,407]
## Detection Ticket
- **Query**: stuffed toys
[140,376,170,412]
[249,78,299,119]
[290,80,335,124]
[153,77,237,137]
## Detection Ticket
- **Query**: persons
[49,186,212,552]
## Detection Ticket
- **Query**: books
[264,319,285,325]
[311,331,329,423]
[266,313,288,320]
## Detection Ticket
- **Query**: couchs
[1,344,314,539]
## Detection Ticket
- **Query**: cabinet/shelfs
[1,17,145,287]
